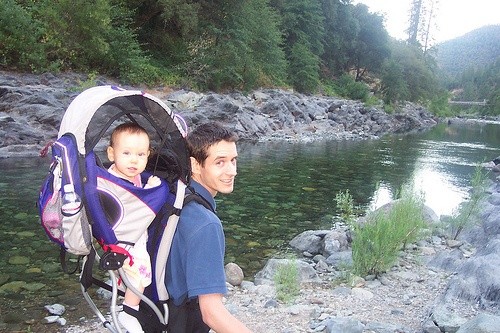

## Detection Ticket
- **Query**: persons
[126,123,253,333]
[107,122,161,333]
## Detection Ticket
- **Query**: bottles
[61,183,83,215]
